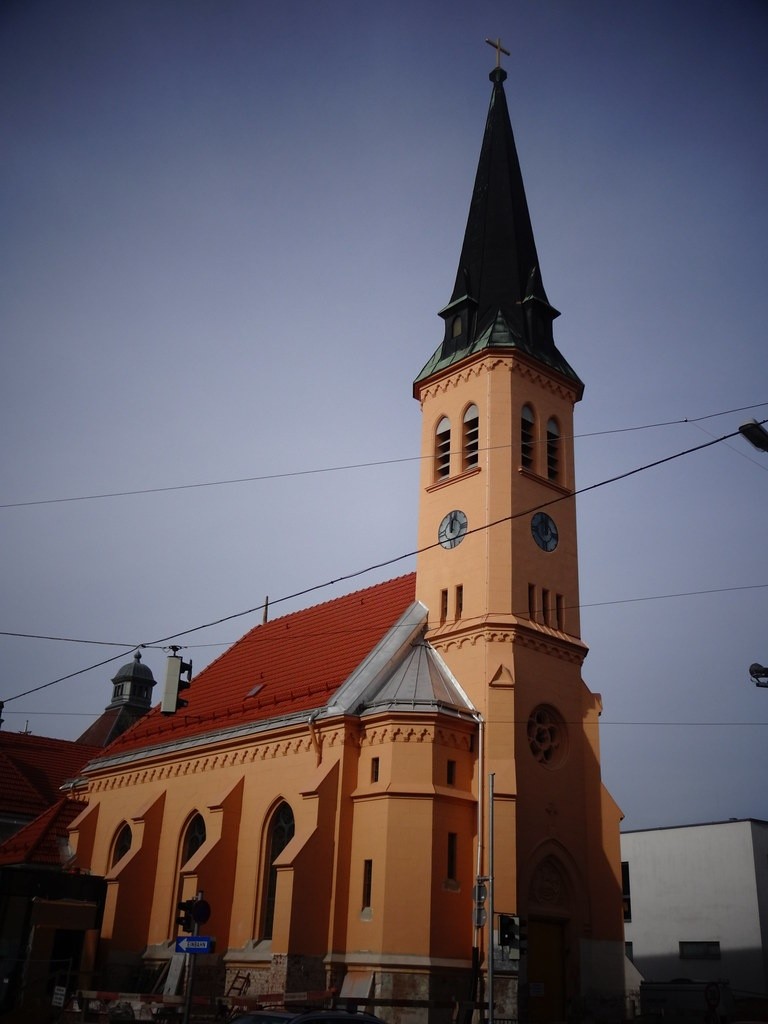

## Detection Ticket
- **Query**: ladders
[213,971,250,1022]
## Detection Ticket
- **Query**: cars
[230,1004,391,1024]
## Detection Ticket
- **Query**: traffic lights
[509,914,529,960]
[161,653,193,716]
[498,913,515,950]
[174,894,211,934]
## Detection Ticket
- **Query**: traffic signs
[175,937,212,954]
